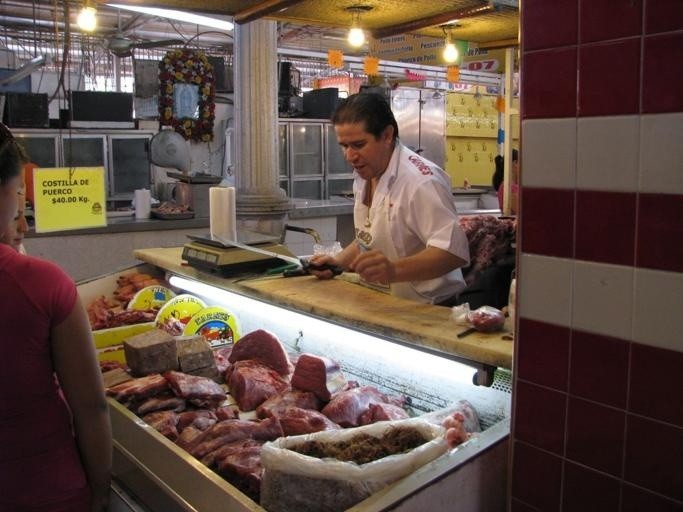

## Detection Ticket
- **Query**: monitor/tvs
[277,62,300,96]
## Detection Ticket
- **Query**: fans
[81,6,185,57]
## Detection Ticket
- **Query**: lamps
[77,0,100,33]
[347,6,366,49]
[438,22,460,65]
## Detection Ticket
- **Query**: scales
[181,231,299,279]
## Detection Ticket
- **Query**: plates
[166,172,223,183]
[151,208,193,219]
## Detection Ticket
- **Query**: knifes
[212,232,343,275]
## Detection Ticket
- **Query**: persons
[491,148,519,217]
[307,92,471,306]
[0,121,113,512]
[0,189,29,252]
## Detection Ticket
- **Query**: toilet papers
[135,191,149,219]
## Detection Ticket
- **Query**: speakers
[2,91,49,129]
